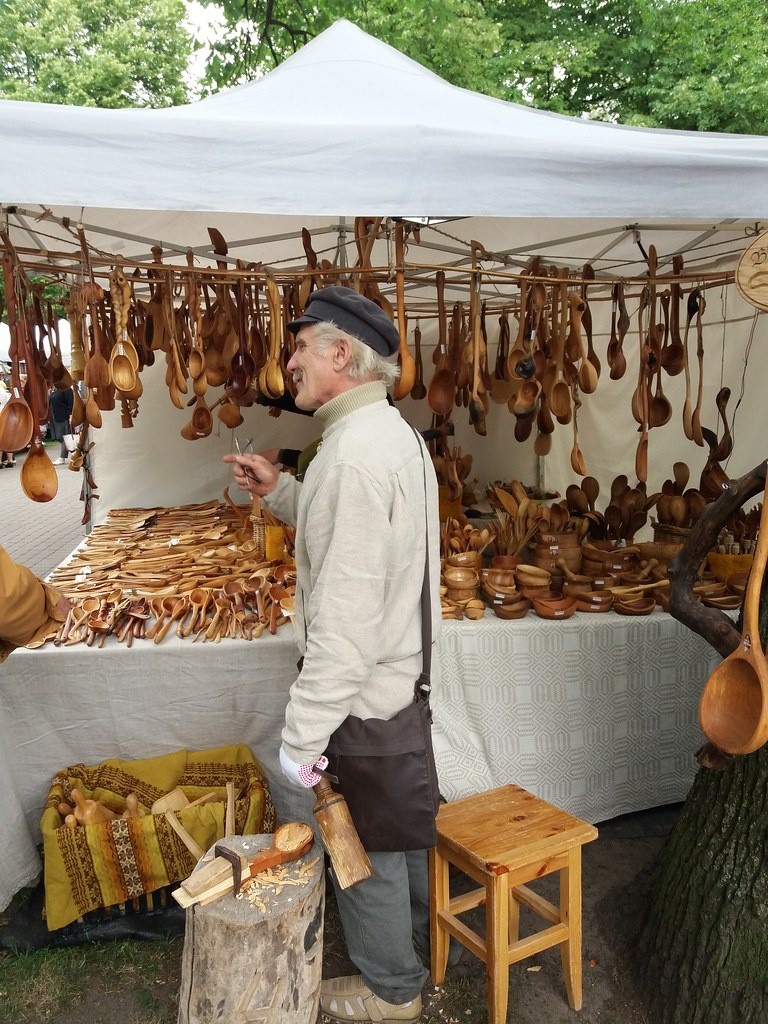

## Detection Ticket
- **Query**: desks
[0,517,740,912]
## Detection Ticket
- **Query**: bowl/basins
[444,531,657,617]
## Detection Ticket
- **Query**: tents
[0,318,72,374]
[0,19,768,545]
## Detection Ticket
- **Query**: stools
[430,782,598,1024]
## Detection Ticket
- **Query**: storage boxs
[42,744,277,948]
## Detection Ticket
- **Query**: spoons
[50,553,302,650]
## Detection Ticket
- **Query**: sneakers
[52,457,65,465]
[321,974,423,1024]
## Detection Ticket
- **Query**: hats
[288,287,401,357]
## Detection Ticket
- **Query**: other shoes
[5,462,13,467]
[0,463,3,469]
[4,459,16,465]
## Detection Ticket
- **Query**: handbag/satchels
[62,424,80,452]
[297,658,442,854]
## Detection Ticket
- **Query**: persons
[222,286,442,1024]
[0,374,82,469]
[0,544,70,662]
